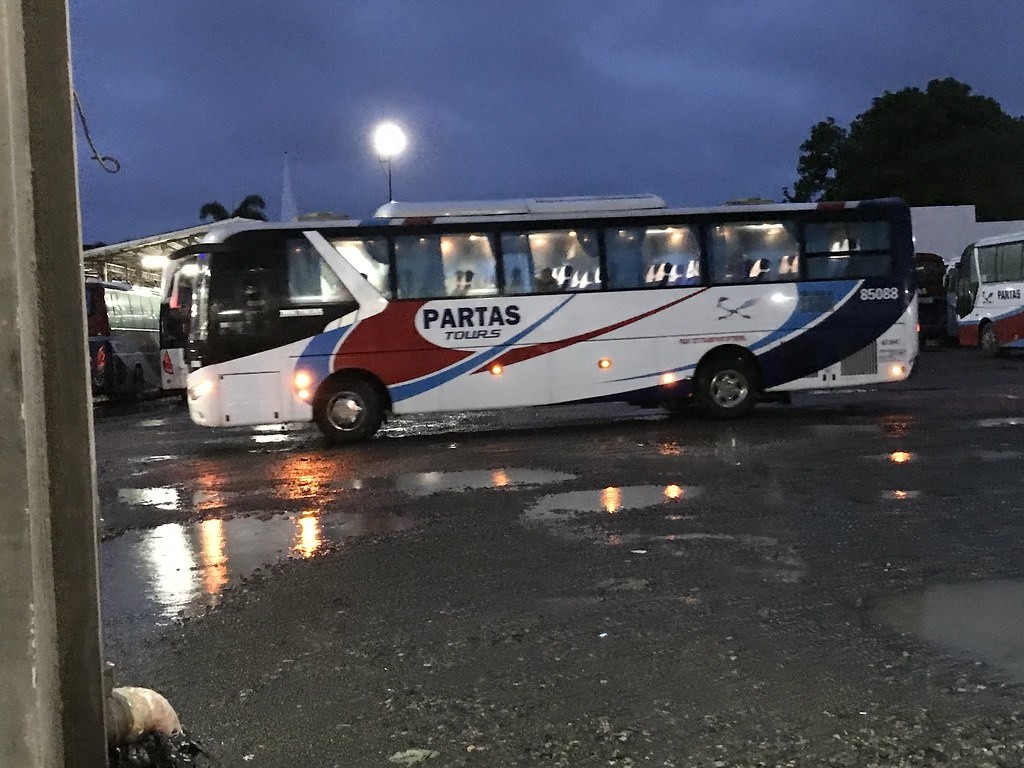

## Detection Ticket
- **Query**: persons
[535,268,559,291]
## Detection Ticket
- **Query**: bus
[84,277,193,408]
[161,191,922,450]
[913,232,1024,358]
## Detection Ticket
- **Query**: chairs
[451,239,849,298]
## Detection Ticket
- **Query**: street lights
[373,122,406,202]
